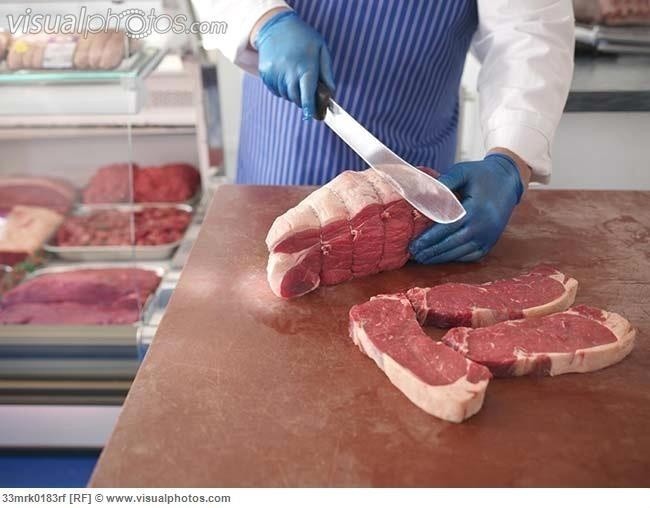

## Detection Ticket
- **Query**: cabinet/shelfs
[0,0,224,454]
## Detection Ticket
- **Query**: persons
[191,0,578,268]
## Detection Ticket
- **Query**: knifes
[233,43,469,229]
